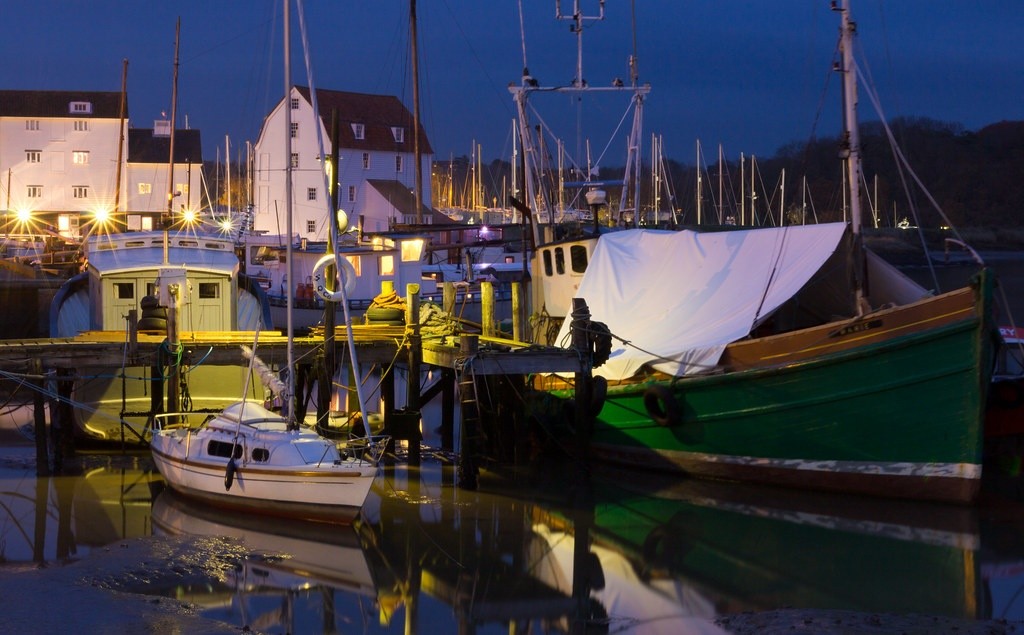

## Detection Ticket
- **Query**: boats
[0,2,1022,635]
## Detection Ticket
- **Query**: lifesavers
[312,253,356,302]
